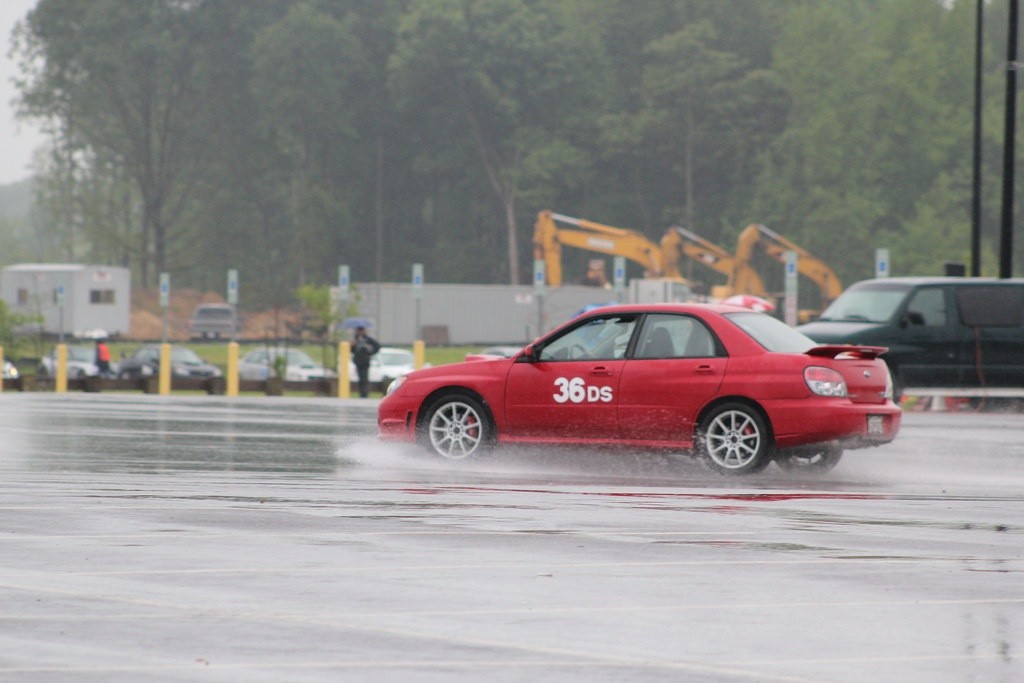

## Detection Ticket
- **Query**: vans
[790,276,1024,390]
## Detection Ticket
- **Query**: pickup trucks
[190,302,240,340]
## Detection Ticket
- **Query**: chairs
[686,325,710,358]
[646,328,675,357]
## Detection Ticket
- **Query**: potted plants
[266,357,286,394]
[18,366,39,390]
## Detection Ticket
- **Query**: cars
[347,347,431,394]
[235,344,337,396]
[117,342,226,394]
[2,358,24,391]
[38,346,104,392]
[376,302,902,477]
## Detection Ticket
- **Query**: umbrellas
[719,293,775,312]
[341,316,372,330]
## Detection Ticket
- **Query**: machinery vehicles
[532,208,841,327]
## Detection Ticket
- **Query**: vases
[143,376,159,394]
[206,378,224,395]
[84,375,101,391]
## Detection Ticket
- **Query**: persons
[348,327,380,397]
[94,342,110,376]
